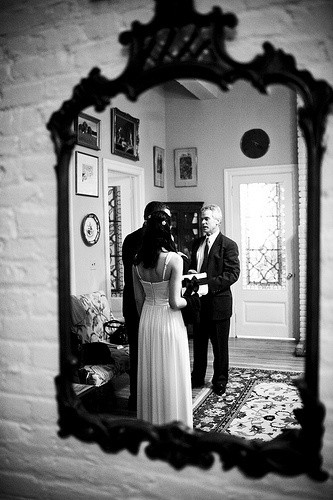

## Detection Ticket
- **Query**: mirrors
[46,0,333,484]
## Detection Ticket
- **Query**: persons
[122,201,200,430]
[184,204,240,395]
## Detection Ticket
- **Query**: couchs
[69,291,132,396]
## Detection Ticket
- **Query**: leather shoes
[214,383,225,394]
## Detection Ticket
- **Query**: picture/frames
[173,147,198,188]
[81,213,100,245]
[153,145,164,188]
[73,112,101,151]
[75,151,99,198]
[111,107,140,162]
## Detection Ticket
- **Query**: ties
[204,238,208,259]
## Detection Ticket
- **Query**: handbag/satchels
[105,320,128,345]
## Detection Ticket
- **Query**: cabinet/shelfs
[160,201,204,256]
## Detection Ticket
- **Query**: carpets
[191,365,305,444]
